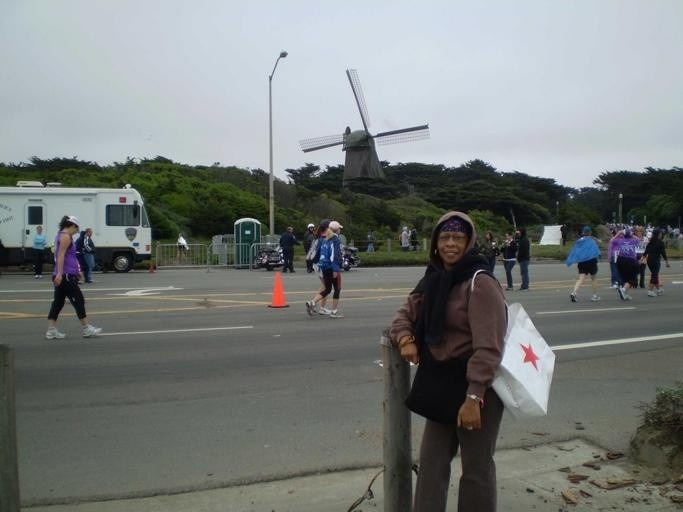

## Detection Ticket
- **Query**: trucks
[0,181,152,273]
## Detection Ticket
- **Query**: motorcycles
[257,246,286,271]
[341,246,360,272]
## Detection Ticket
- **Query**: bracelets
[397,335,415,350]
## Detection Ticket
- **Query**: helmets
[308,223,316,233]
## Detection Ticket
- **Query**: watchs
[465,394,482,404]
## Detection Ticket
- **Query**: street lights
[556,201,559,218]
[268,49,290,234]
[617,193,624,223]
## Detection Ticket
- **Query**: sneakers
[45,329,67,340]
[658,290,664,295]
[81,325,103,337]
[648,291,657,298]
[305,300,316,317]
[330,310,344,318]
[318,307,331,314]
[34,273,42,278]
[570,292,578,303]
[623,294,633,301]
[618,287,627,300]
[590,295,602,301]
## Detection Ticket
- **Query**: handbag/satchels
[491,302,556,422]
[404,350,476,425]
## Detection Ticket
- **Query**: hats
[320,219,332,227]
[329,220,343,230]
[64,215,81,233]
[583,226,592,233]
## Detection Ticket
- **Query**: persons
[33,216,102,339]
[390,210,508,512]
[479,232,499,272]
[176,233,189,259]
[279,218,345,318]
[516,228,529,291]
[566,222,683,303]
[401,227,411,251]
[499,233,517,289]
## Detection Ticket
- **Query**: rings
[466,426,472,430]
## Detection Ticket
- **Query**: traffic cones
[267,271,291,308]
